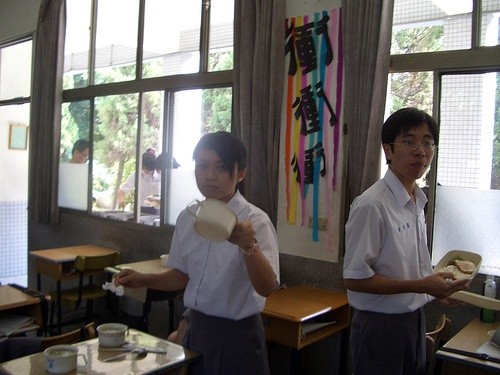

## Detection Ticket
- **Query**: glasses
[389,139,436,152]
[79,150,88,159]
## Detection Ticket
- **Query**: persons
[117,146,161,209]
[111,131,280,375]
[343,107,470,375]
[67,139,96,207]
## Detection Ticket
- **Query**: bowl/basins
[160,254,169,266]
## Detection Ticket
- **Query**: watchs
[241,239,259,256]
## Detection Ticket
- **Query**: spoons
[103,348,147,362]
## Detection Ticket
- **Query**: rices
[438,265,471,281]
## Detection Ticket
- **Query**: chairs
[426,313,450,375]
[0,323,96,366]
[50,251,120,327]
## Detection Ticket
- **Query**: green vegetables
[152,194,161,196]
[453,256,464,261]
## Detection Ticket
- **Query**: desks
[0,244,500,375]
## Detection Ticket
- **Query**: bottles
[480,274,497,323]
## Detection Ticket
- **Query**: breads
[455,260,476,273]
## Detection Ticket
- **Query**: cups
[96,323,130,348]
[43,345,89,375]
[186,198,238,242]
